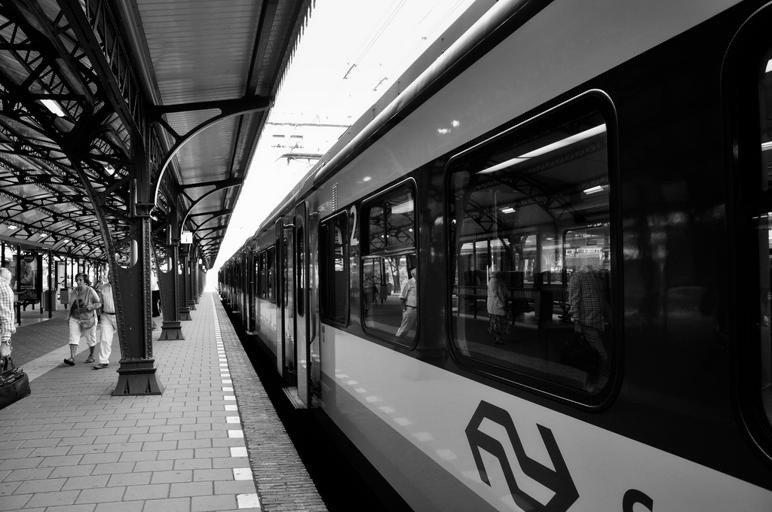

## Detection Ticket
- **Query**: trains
[217,0,772,512]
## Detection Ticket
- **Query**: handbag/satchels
[0,356,32,411]
[79,316,95,329]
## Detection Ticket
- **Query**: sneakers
[92,364,109,369]
[86,354,96,363]
[64,358,75,366]
[152,309,162,317]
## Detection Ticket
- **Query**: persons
[395,266,417,338]
[370,283,378,305]
[379,280,389,305]
[525,270,535,284]
[94,271,119,369]
[0,268,12,284]
[95,275,103,289]
[566,244,610,385]
[0,277,16,359]
[63,273,102,366]
[487,255,516,350]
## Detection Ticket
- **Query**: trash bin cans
[60,288,69,304]
[44,289,56,311]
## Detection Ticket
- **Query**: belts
[103,311,116,315]
